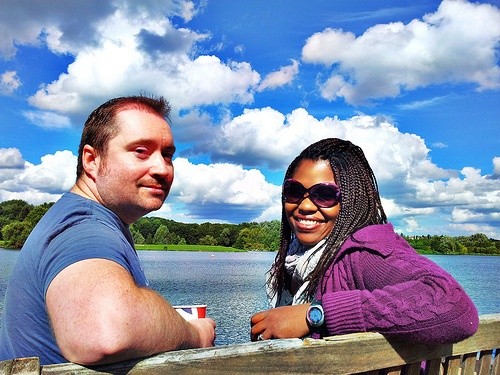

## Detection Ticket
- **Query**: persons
[251,138,479,375]
[0,96,217,366]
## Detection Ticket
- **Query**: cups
[172,305,207,321]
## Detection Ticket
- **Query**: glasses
[282,179,341,208]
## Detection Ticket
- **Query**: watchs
[306,299,325,333]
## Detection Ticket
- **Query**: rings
[257,334,264,340]
